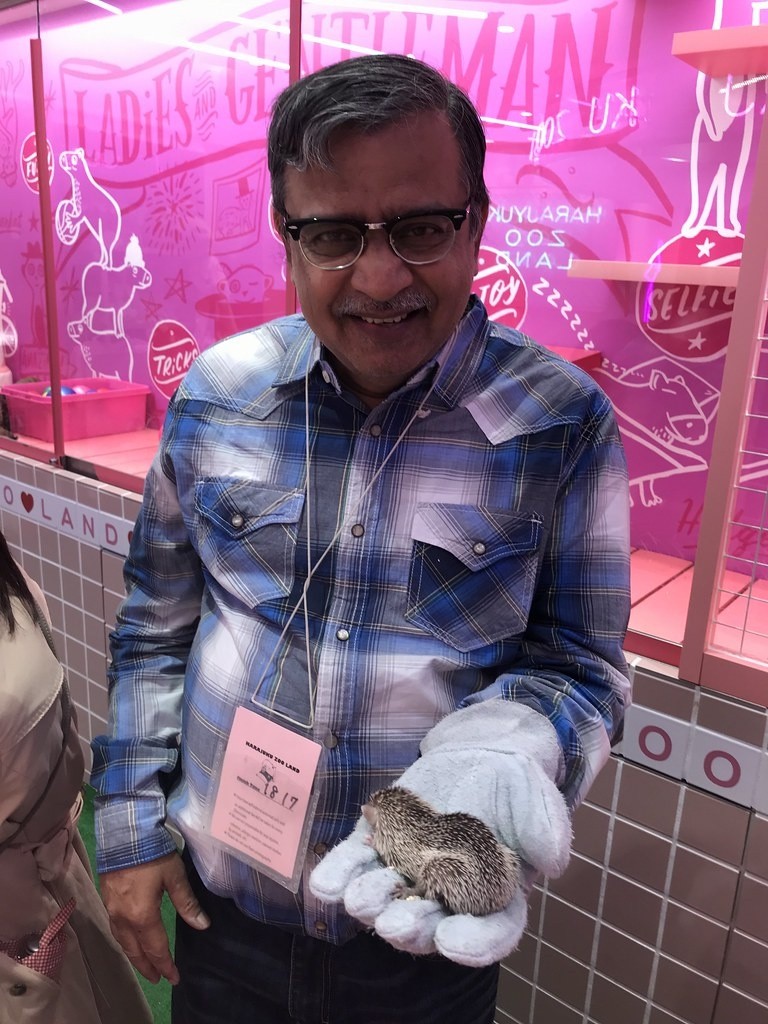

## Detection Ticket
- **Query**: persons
[0,535,155,1024]
[93,54,635,1024]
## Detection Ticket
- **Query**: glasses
[283,193,471,270]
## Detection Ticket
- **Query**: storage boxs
[0,376,152,443]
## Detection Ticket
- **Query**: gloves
[309,699,574,968]
